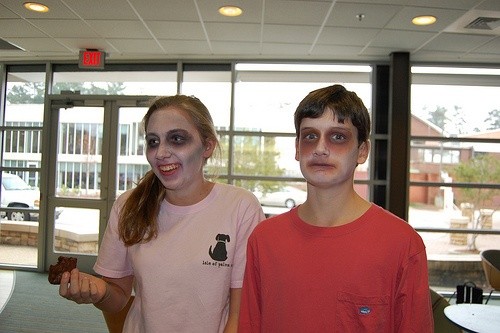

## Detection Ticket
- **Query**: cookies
[48,256,78,285]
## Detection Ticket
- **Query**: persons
[58,94,266,333]
[237,83,434,333]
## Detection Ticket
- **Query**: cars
[252,185,307,208]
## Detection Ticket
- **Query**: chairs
[481,250,500,305]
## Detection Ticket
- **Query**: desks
[444,304,500,333]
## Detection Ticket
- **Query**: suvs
[0,173,63,222]
